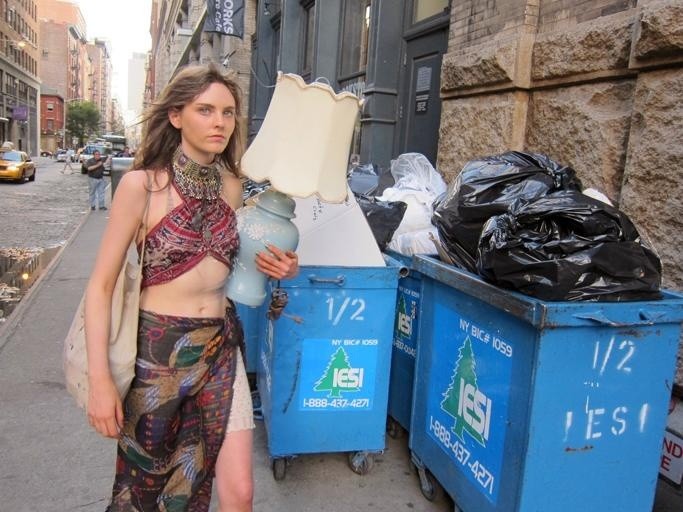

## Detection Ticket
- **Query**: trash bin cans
[384,244,424,440]
[253,251,411,481]
[407,252,683,512]
[233,278,272,376]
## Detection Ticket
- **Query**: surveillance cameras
[222,56,231,67]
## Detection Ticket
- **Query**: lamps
[226,70,366,308]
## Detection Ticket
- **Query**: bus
[100,132,125,152]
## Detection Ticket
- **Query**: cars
[81,145,110,175]
[16,151,35,184]
[40,148,81,162]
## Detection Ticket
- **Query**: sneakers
[90,206,95,211]
[98,206,108,210]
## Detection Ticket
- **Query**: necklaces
[173,144,224,201]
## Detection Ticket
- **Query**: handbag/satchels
[60,242,145,418]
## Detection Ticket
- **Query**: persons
[60,148,74,175]
[3,139,13,147]
[118,147,131,157]
[83,150,111,209]
[83,62,298,512]
